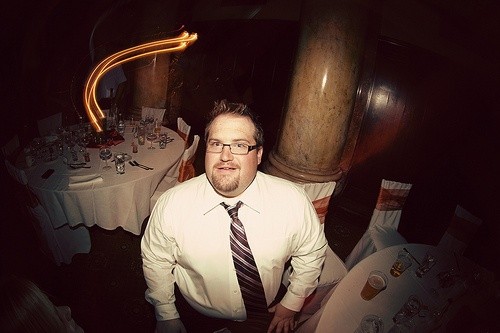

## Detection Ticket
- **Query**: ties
[220,201,271,333]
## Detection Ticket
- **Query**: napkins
[68,173,103,186]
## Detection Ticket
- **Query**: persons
[140,99,328,333]
[0,275,84,333]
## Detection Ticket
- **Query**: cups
[354,314,385,333]
[114,151,126,174]
[390,250,413,278]
[387,294,448,333]
[360,271,388,301]
[155,120,162,133]
[129,115,135,125]
[415,254,435,277]
[159,134,167,149]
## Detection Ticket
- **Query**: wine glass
[144,126,157,149]
[99,145,112,170]
[31,124,92,166]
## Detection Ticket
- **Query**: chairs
[345,180,412,273]
[282,182,347,314]
[176,117,191,145]
[0,113,91,266]
[440,204,486,259]
[142,106,166,124]
[178,135,200,184]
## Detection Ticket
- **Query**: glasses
[204,142,259,155]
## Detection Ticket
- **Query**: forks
[70,165,91,169]
[128,160,153,170]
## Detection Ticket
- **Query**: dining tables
[11,120,186,237]
[296,243,500,333]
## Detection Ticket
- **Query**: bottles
[118,114,124,133]
